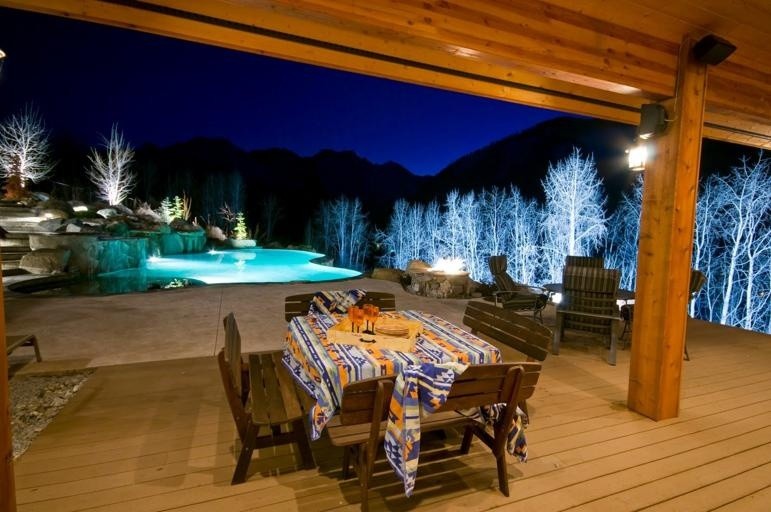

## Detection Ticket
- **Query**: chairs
[489,253,549,327]
[552,254,704,366]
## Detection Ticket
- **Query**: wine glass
[348,304,379,335]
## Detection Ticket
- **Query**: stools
[5,334,42,379]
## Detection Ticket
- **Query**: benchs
[285,291,396,324]
[216,311,320,485]
[327,361,542,511]
[462,300,553,427]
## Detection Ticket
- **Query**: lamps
[626,143,646,172]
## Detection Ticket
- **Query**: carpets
[10,368,99,463]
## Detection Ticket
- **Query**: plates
[375,324,408,335]
